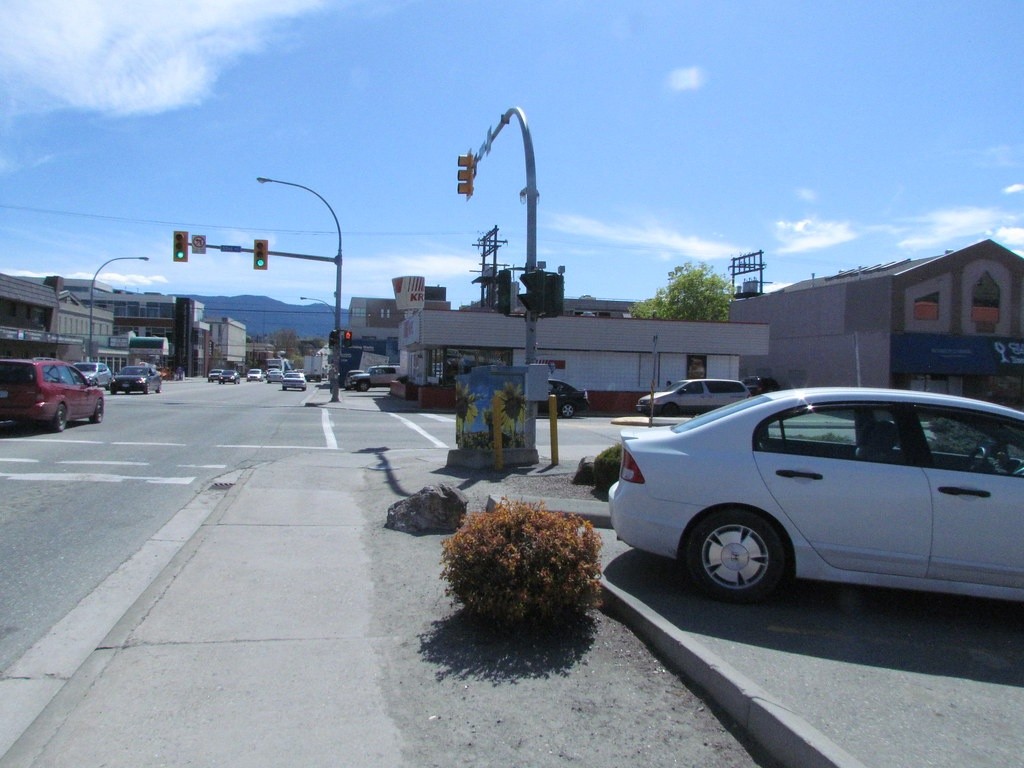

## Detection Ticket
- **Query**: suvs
[741,376,780,396]
[635,379,752,417]
[0,357,105,433]
[350,363,400,392]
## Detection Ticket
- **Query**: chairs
[848,419,905,465]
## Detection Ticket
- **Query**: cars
[72,363,113,390]
[110,365,162,396]
[208,368,307,391]
[345,370,364,390]
[538,379,590,417]
[609,386,1024,605]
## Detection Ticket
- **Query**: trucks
[303,356,322,382]
[265,359,284,374]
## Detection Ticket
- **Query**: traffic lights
[174,230,188,262]
[458,155,468,194]
[517,273,541,312]
[344,332,351,347]
[253,240,268,270]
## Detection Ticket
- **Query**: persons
[666,381,671,387]
[328,365,335,393]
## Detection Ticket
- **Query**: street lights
[89,257,149,361]
[220,319,251,369]
[257,177,343,402]
